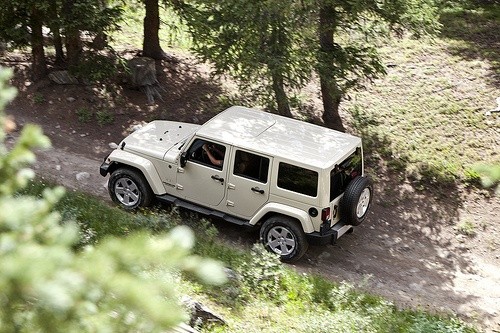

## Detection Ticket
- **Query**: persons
[202,144,244,172]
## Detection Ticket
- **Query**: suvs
[100,106,373,264]
